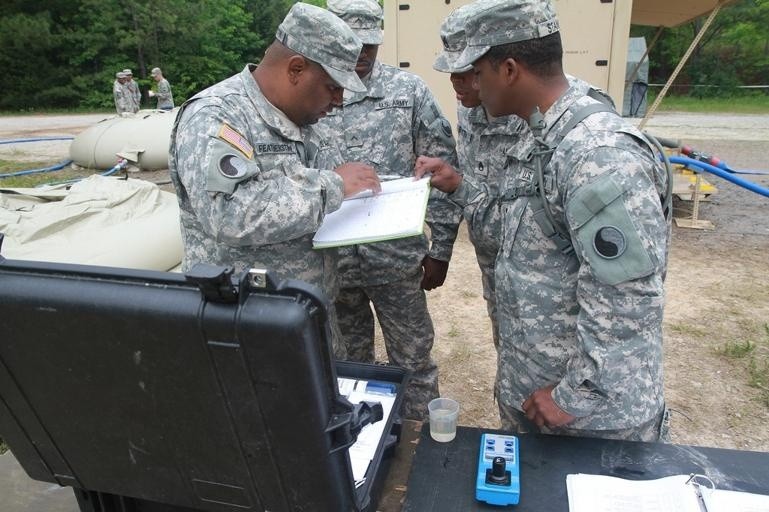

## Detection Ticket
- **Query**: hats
[116,69,133,79]
[276,1,384,94]
[452,0,562,69]
[432,3,474,73]
[151,67,161,78]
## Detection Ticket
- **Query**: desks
[0,419,769,512]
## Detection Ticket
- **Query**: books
[310,173,435,250]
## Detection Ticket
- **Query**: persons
[169,0,384,365]
[112,71,140,115]
[149,67,175,109]
[121,69,143,110]
[432,1,622,362]
[302,0,464,420]
[408,0,671,444]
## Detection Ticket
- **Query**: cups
[428,398,459,443]
